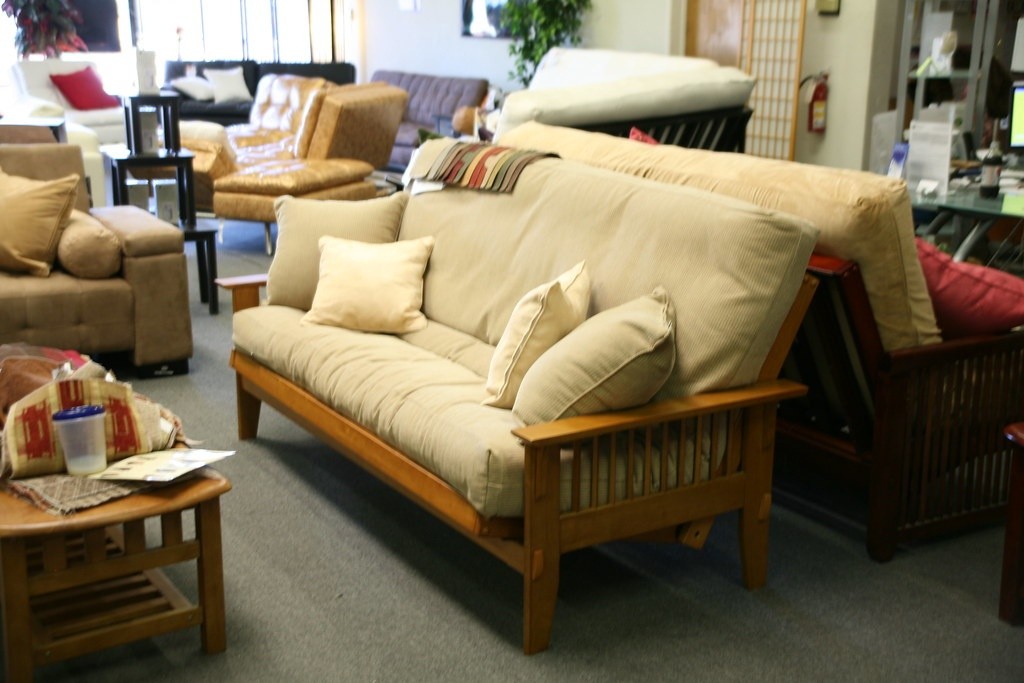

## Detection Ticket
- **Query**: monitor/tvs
[1008,82,1024,152]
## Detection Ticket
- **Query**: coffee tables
[0,443,232,683]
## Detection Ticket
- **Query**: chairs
[9,55,126,144]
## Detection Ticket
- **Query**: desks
[908,186,1024,262]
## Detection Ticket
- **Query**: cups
[52,405,108,477]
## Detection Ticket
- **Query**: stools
[99,92,221,316]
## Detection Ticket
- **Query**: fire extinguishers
[799,72,831,135]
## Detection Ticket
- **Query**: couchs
[0,140,194,378]
[488,44,758,154]
[156,74,406,254]
[213,138,818,655]
[369,68,488,166]
[502,121,1024,562]
[160,57,356,127]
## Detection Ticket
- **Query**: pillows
[483,260,591,409]
[302,235,432,334]
[508,285,676,449]
[170,75,213,101]
[49,66,119,110]
[0,166,80,277]
[914,235,1024,339]
[266,190,409,311]
[57,208,121,279]
[203,64,254,105]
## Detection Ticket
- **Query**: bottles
[980,140,1003,199]
